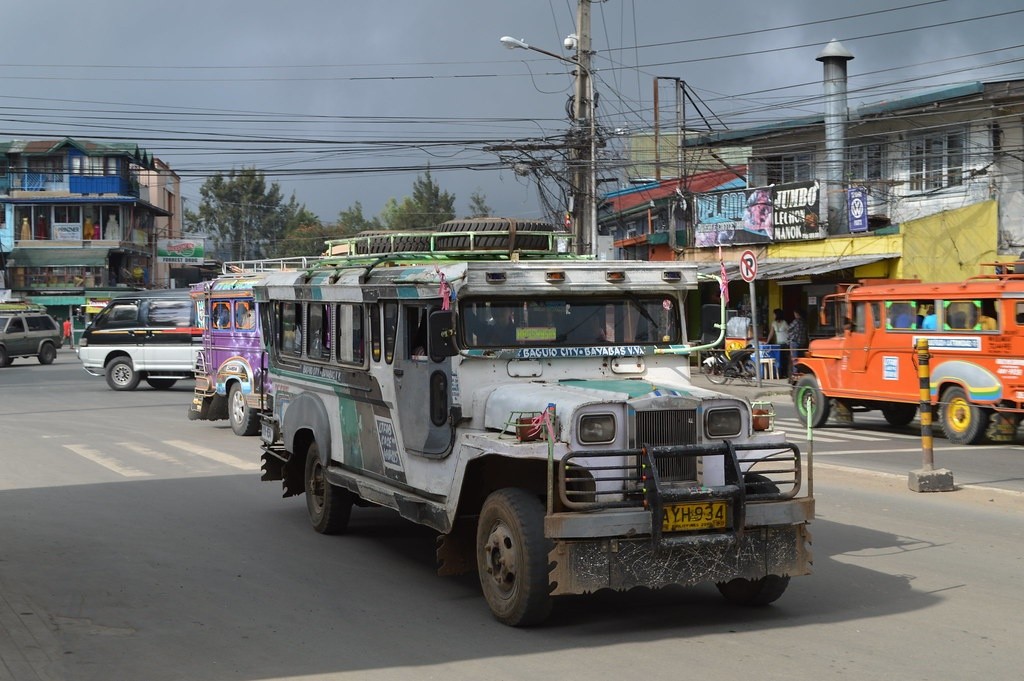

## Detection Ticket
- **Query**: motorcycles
[696,345,758,387]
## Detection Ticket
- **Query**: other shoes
[781,376,787,379]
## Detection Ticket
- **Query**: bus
[188,255,503,438]
[790,260,1024,442]
[257,252,822,629]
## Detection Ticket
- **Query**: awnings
[690,255,905,281]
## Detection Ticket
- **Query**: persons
[890,304,937,330]
[762,308,806,378]
[85,213,118,240]
[37,215,47,240]
[20,217,30,240]
[60,316,74,349]
[486,299,521,347]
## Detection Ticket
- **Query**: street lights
[496,36,598,259]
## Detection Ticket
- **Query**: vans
[76,292,197,390]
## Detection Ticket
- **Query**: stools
[759,358,779,380]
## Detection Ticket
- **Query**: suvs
[0,306,63,369]
